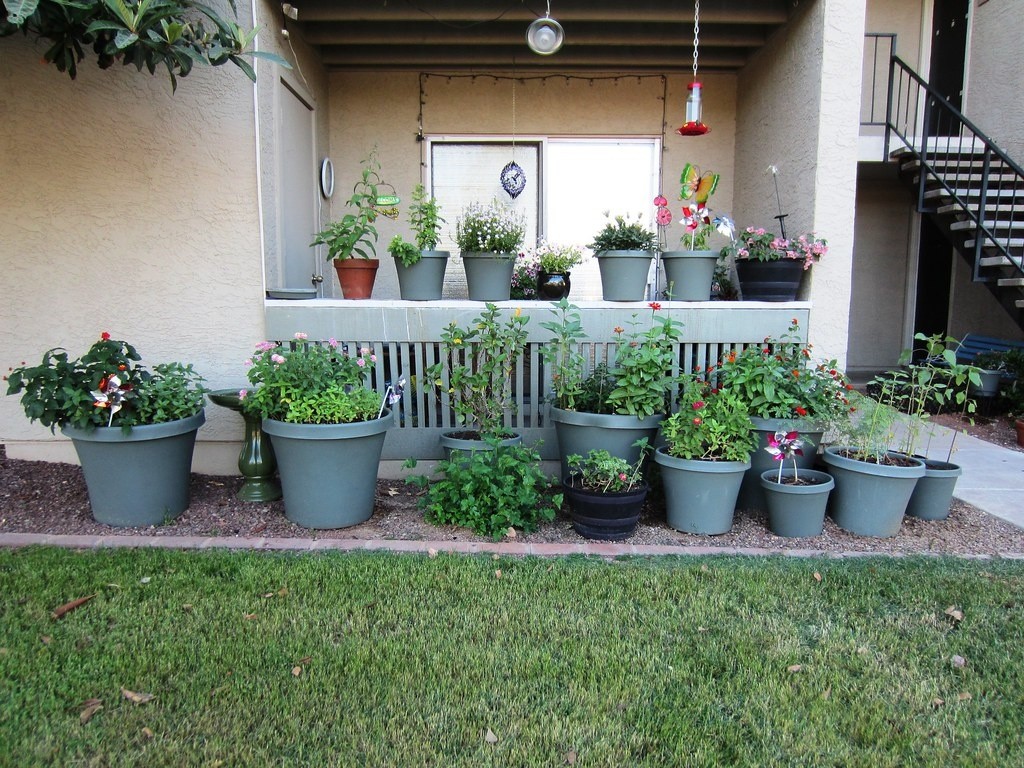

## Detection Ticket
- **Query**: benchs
[916,335,1023,397]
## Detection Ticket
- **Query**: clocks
[322,157,335,198]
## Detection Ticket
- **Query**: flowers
[1,332,209,437]
[453,200,539,303]
[532,236,583,275]
[717,217,829,270]
[238,334,383,424]
[538,281,860,480]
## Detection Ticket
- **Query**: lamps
[525,0,565,56]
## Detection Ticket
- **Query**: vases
[760,468,834,538]
[535,270,570,300]
[61,404,204,527]
[1015,418,1024,448]
[210,387,287,501]
[655,446,753,535]
[262,407,393,531]
[460,250,517,301]
[569,471,650,540]
[548,404,664,476]
[732,257,804,303]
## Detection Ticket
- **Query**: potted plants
[422,300,533,475]
[386,183,450,300]
[587,208,656,303]
[308,140,396,300]
[822,330,981,538]
[968,347,1003,397]
[657,223,718,302]
